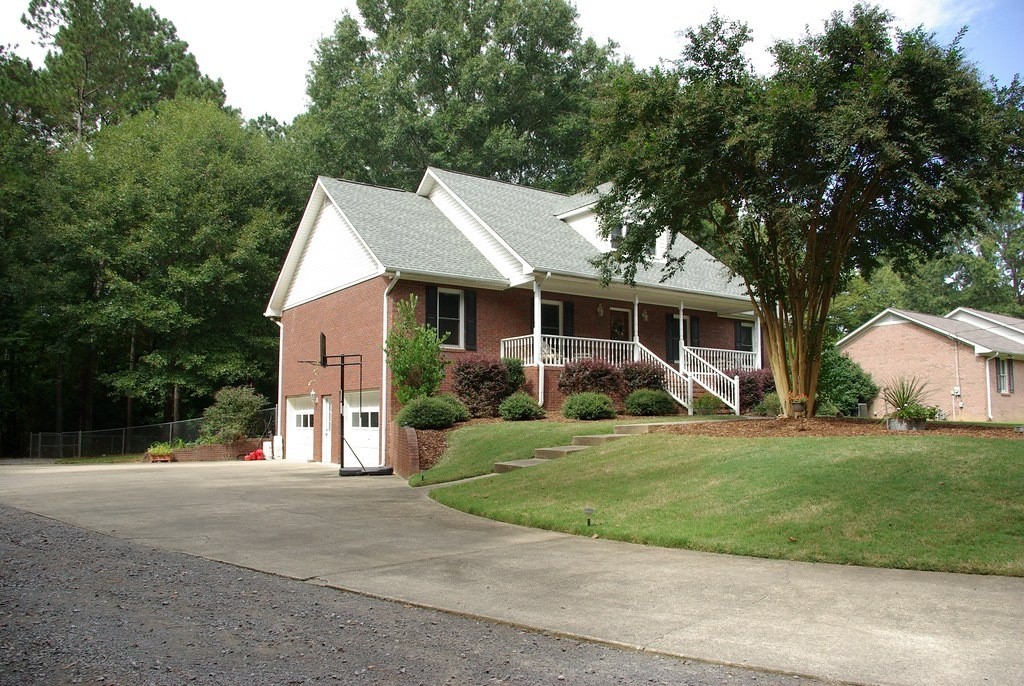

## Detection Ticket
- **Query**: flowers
[787,391,809,402]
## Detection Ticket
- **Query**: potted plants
[145,444,175,463]
[876,373,939,432]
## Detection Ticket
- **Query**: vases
[791,403,806,412]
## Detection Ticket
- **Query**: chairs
[542,341,563,364]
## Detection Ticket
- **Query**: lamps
[310,388,319,404]
[642,308,649,321]
[597,304,604,316]
